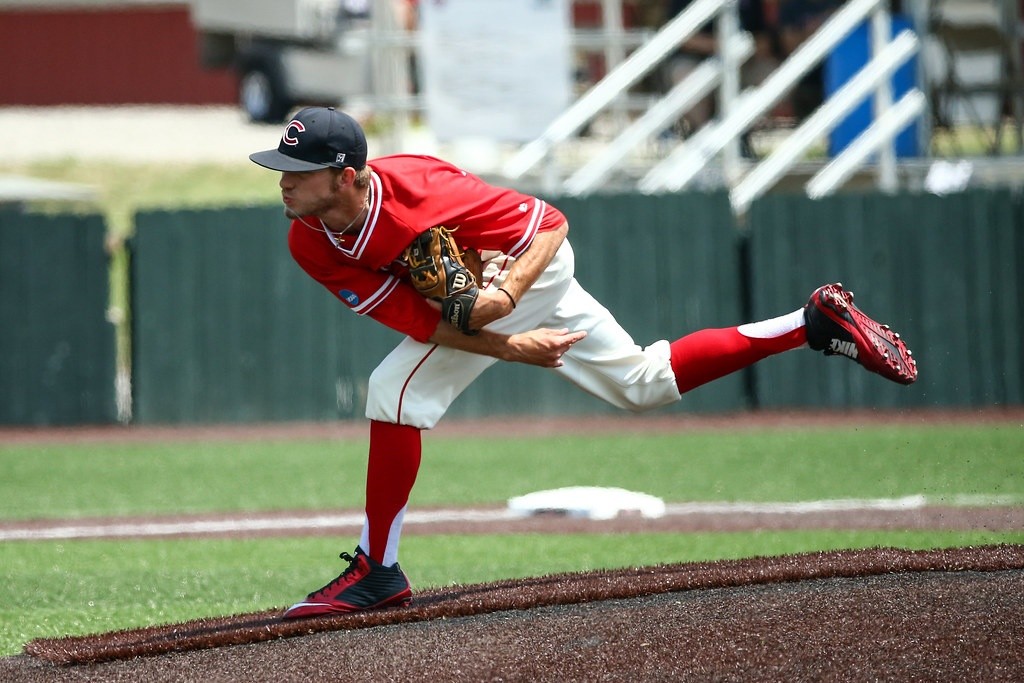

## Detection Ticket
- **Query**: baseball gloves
[401,227,479,336]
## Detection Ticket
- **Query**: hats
[248,106,367,171]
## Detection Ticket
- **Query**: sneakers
[804,283,918,384]
[282,544,413,617]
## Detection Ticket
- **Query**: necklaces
[286,206,366,248]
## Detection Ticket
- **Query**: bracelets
[498,288,516,308]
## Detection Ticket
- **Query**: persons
[249,107,918,620]
